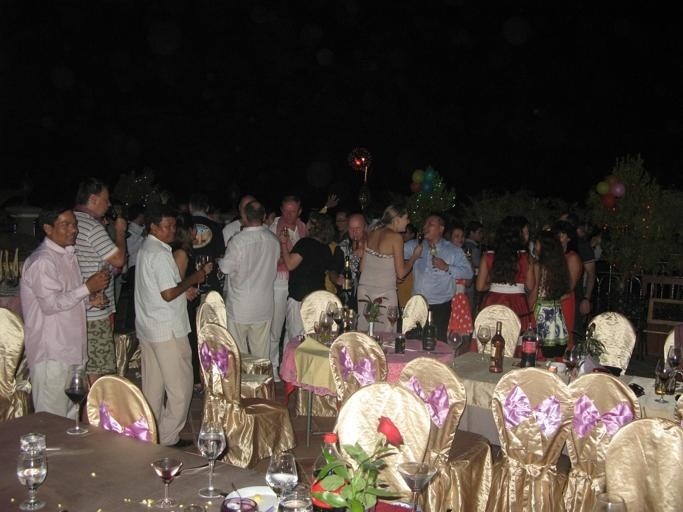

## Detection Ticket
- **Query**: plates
[225,485,286,511]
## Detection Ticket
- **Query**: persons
[171,209,203,396]
[319,192,340,212]
[73,182,127,419]
[559,213,597,340]
[337,214,367,312]
[442,225,473,335]
[220,193,262,243]
[335,212,349,234]
[477,218,536,359]
[405,212,476,343]
[526,230,572,357]
[357,205,423,332]
[183,186,228,291]
[550,221,581,349]
[21,204,111,419]
[280,213,336,349]
[135,204,206,448]
[269,192,307,381]
[461,221,483,318]
[218,201,280,358]
[127,208,151,328]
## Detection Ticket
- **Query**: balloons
[421,180,432,193]
[611,182,626,199]
[602,192,616,208]
[597,181,610,196]
[411,182,421,193]
[412,169,424,183]
[606,174,619,188]
[424,168,436,181]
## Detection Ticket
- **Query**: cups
[430,246,437,270]
[97,261,111,308]
[15,432,46,511]
[333,307,347,335]
[264,452,299,500]
[221,498,257,511]
[591,491,628,512]
[477,325,492,362]
[195,256,203,293]
[363,303,371,338]
[563,344,580,376]
[280,482,311,498]
[347,309,356,330]
[417,232,424,259]
[397,461,438,512]
[197,421,227,499]
[385,305,400,338]
[325,300,338,318]
[447,329,463,369]
[202,255,210,287]
[150,458,183,509]
[180,503,206,512]
[666,344,682,369]
[63,364,90,436]
[277,494,313,511]
[654,358,671,404]
[320,310,332,338]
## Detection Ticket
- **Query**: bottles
[395,335,406,354]
[520,322,536,369]
[309,433,351,512]
[489,321,505,374]
[343,256,355,292]
[423,309,436,351]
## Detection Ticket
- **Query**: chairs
[491,367,574,512]
[193,321,297,469]
[605,417,682,512]
[400,292,429,333]
[299,289,343,334]
[567,374,642,512]
[0,308,31,419]
[473,304,522,359]
[196,302,276,398]
[313,380,439,504]
[586,311,637,375]
[204,289,272,375]
[86,375,158,444]
[326,330,387,415]
[399,353,493,512]
